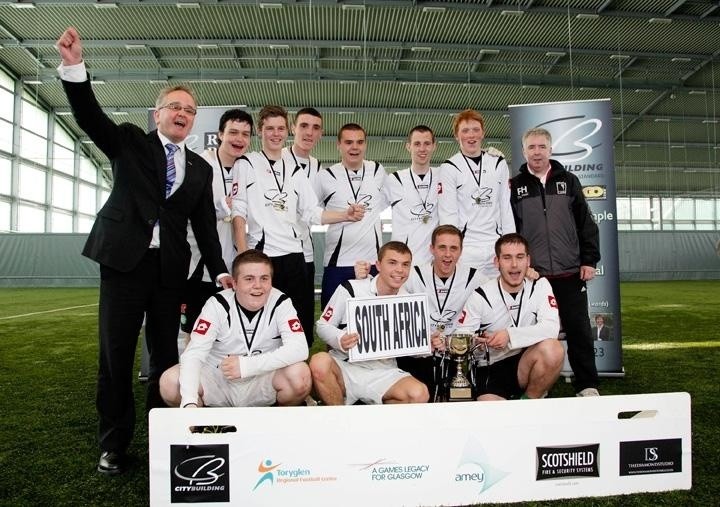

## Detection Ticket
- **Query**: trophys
[438,332,491,402]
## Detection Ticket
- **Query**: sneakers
[576,388,600,397]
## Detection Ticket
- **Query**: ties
[153,143,180,225]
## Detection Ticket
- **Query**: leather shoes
[97,451,124,473]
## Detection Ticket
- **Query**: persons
[159,250,312,408]
[380,126,440,266]
[55,28,233,474]
[313,123,388,330]
[355,225,539,402]
[181,108,253,343]
[232,104,364,346]
[590,315,609,342]
[437,110,517,280]
[308,242,444,404]
[508,128,601,396]
[434,233,565,400]
[279,108,323,203]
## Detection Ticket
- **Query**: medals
[475,196,481,204]
[438,325,445,332]
[422,215,429,224]
[278,202,285,211]
[223,216,231,223]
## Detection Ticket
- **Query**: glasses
[158,103,196,115]
